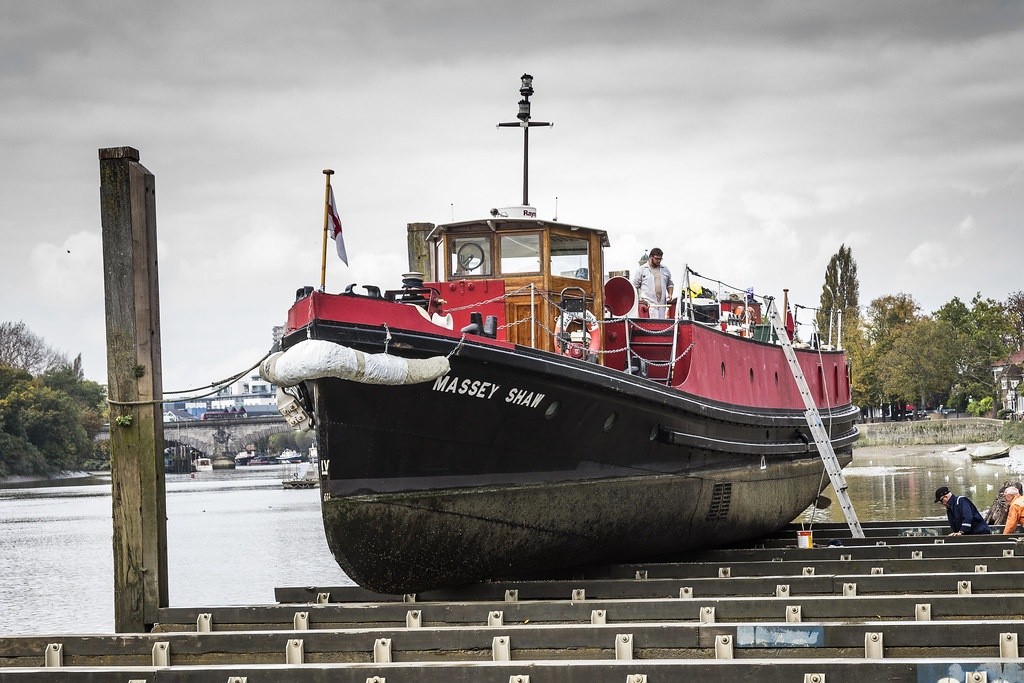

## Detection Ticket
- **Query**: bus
[200,411,245,420]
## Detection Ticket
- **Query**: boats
[194,458,213,472]
[279,72,861,595]
[246,458,270,465]
[275,448,302,464]
[969,444,1016,461]
[234,451,255,466]
[308,442,318,463]
[212,456,236,469]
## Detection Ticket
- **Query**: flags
[323,184,349,266]
[785,302,795,342]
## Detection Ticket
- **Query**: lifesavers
[554,310,601,363]
[733,306,756,339]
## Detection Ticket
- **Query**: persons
[933,487,992,537]
[632,248,674,319]
[1002,487,1024,535]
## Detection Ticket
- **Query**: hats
[934,487,951,503]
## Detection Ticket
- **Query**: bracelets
[958,531,964,534]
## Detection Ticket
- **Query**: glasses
[940,496,945,503]
[652,255,663,261]
